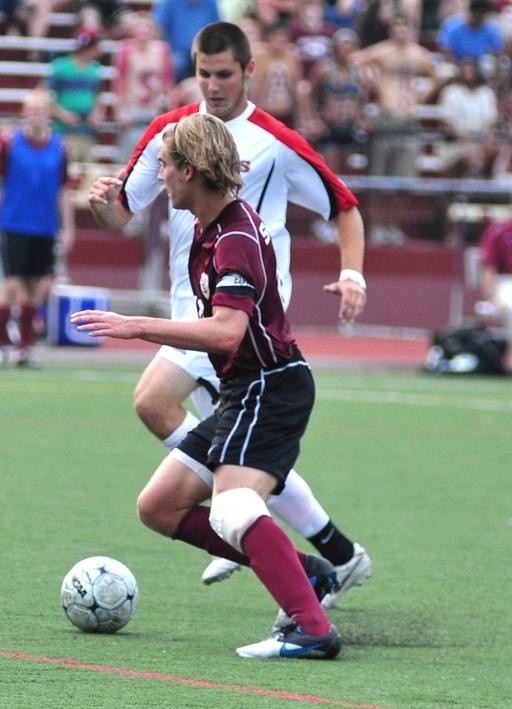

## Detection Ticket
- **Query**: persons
[87,19,371,609]
[68,113,342,659]
[0,84,79,366]
[1,1,511,245]
[473,217,510,370]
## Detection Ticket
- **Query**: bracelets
[338,265,366,288]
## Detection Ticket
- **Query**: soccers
[61,556,139,633]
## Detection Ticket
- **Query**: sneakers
[321,540,374,610]
[271,552,341,635]
[200,558,242,588]
[235,621,343,660]
